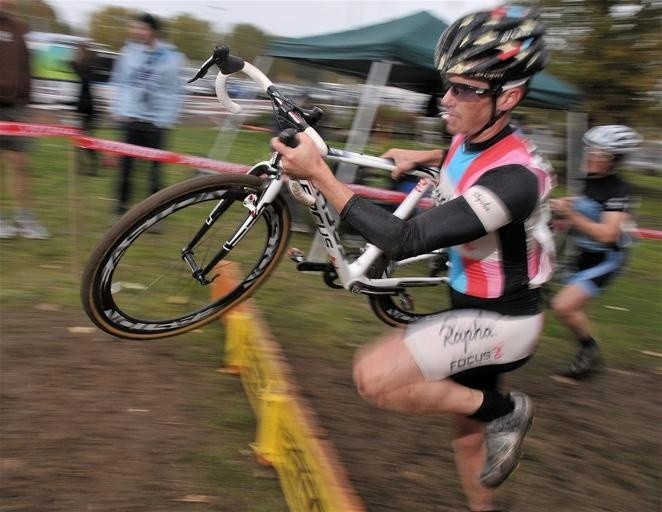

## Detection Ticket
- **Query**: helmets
[583,125,644,153]
[434,6,548,82]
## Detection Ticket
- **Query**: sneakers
[480,391,535,488]
[555,345,603,380]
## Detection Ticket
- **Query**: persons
[2,0,53,242]
[269,2,557,510]
[270,86,318,234]
[107,10,190,233]
[64,40,100,178]
[545,123,646,390]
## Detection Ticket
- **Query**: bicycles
[81,46,569,340]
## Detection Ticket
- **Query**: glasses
[441,75,499,103]
